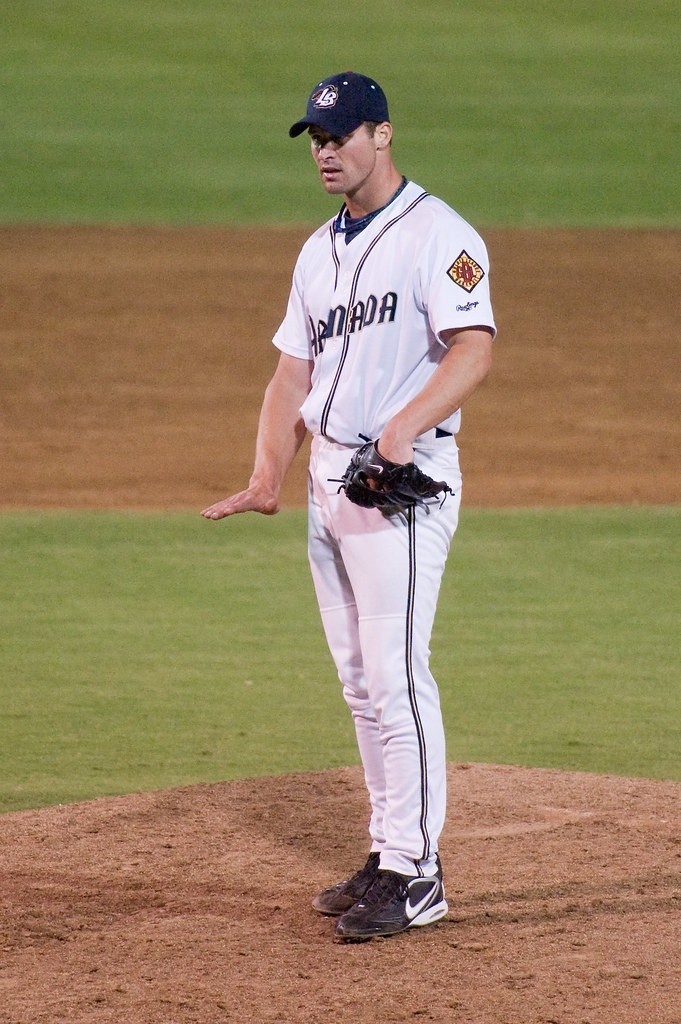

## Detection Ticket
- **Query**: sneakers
[310,850,385,916]
[334,851,449,940]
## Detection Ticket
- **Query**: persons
[198,71,498,942]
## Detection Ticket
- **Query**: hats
[288,70,389,139]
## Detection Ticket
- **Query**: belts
[434,425,453,438]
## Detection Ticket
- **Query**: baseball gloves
[325,430,456,528]
